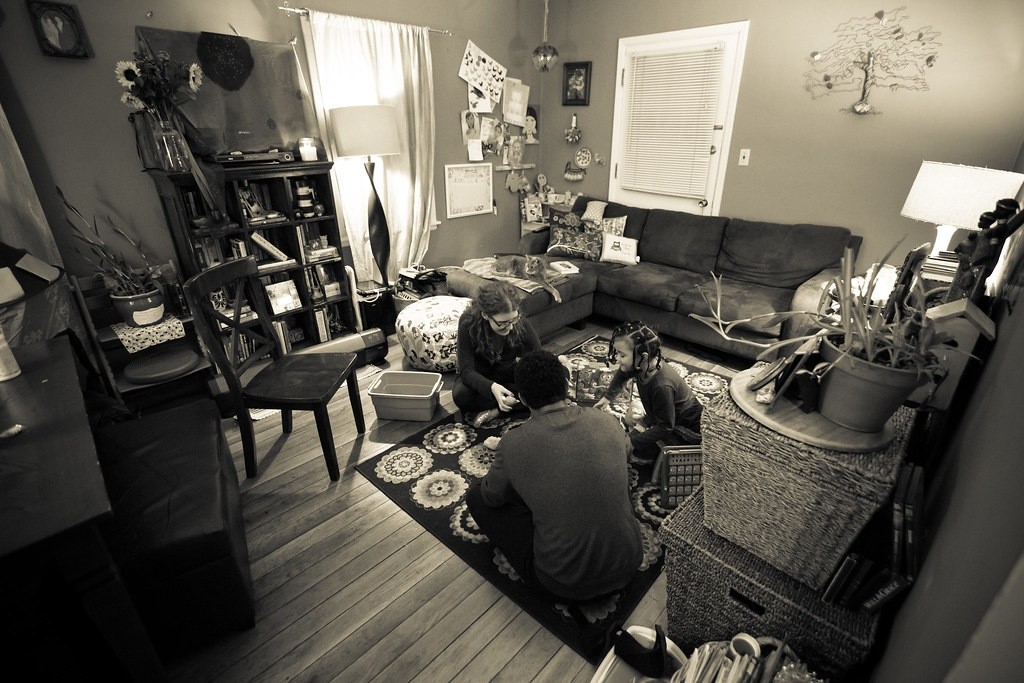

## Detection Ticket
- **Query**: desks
[0,308,165,680]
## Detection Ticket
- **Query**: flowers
[113,49,202,165]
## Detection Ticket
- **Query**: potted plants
[53,184,167,328]
[687,228,988,426]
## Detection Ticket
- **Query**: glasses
[486,310,521,327]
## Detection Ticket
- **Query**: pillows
[546,201,640,266]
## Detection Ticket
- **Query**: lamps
[900,160,1024,256]
[326,104,403,291]
[532,0,560,72]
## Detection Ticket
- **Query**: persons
[468,350,645,603]
[592,321,704,458]
[452,280,543,427]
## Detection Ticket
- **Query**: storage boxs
[367,370,445,423]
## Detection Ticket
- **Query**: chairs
[182,255,362,483]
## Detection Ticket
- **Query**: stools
[395,295,472,369]
[444,262,597,339]
[53,398,259,649]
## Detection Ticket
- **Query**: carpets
[354,333,734,666]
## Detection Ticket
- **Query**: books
[550,260,579,274]
[675,642,759,683]
[184,180,331,376]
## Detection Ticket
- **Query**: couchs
[518,196,863,362]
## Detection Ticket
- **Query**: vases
[151,121,194,174]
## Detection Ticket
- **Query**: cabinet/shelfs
[144,157,354,377]
[68,260,215,411]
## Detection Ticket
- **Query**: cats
[490,255,563,303]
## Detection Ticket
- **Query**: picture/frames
[562,61,591,106]
[25,0,94,60]
